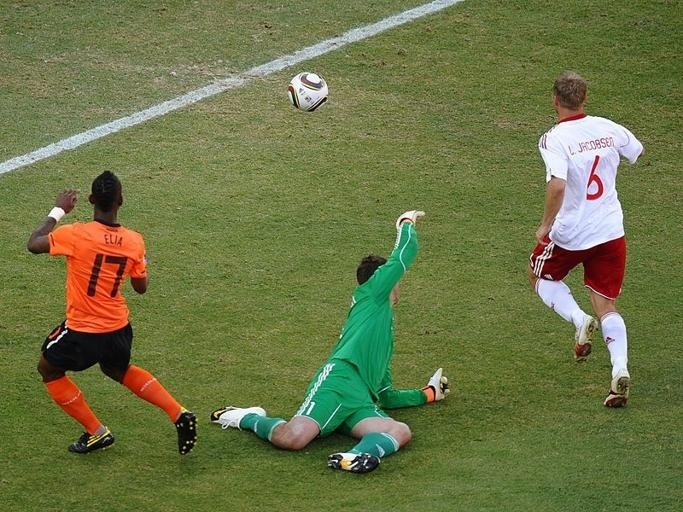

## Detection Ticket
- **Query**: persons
[213,208,450,474]
[529,69,645,407]
[26,171,197,455]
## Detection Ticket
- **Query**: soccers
[287,72,328,112]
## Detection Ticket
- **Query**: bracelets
[144,257,148,266]
[48,206,66,222]
[420,386,435,403]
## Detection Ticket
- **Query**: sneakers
[210,406,267,430]
[573,315,599,364]
[603,369,631,409]
[327,452,380,474]
[174,407,199,456]
[67,426,116,454]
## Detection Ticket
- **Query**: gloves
[421,367,451,403]
[395,209,426,232]
